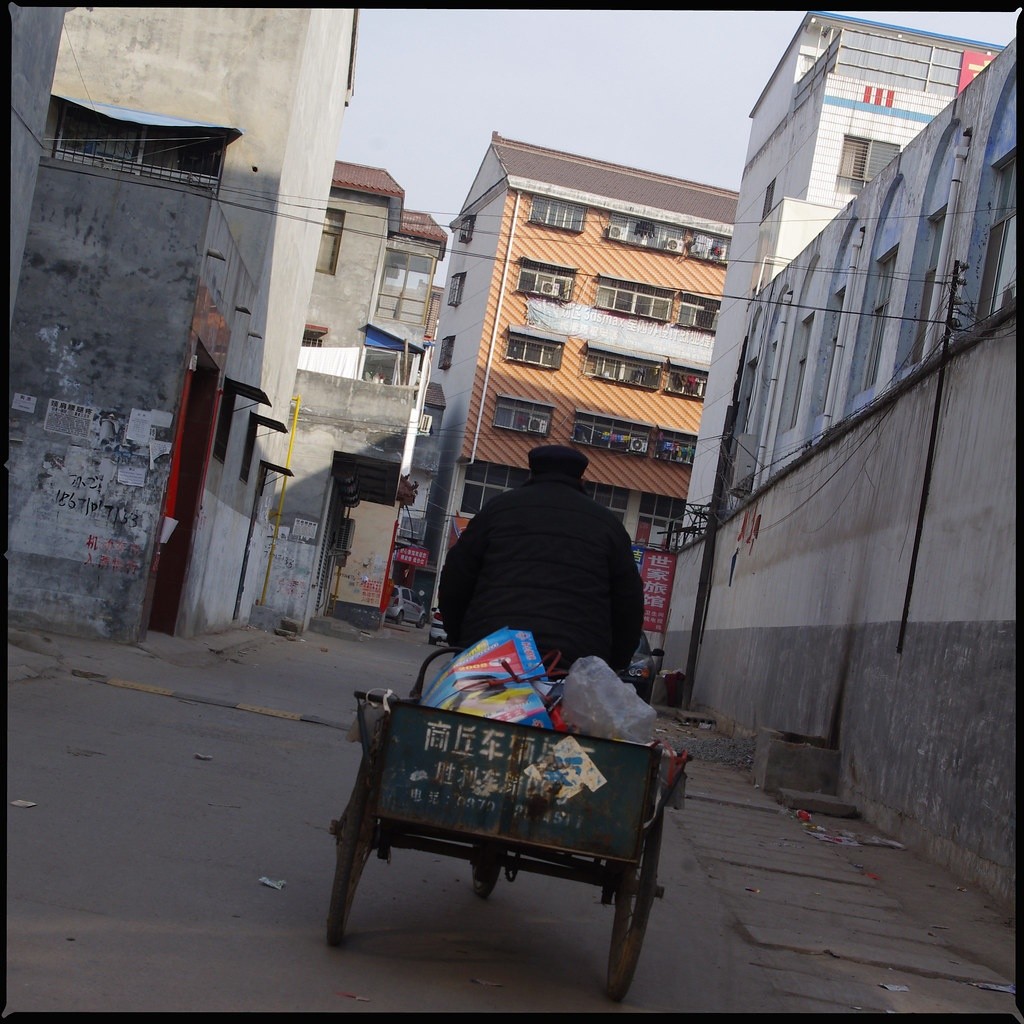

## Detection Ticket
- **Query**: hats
[528,445,589,477]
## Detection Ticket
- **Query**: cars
[385,583,428,629]
[428,605,448,646]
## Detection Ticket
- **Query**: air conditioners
[608,225,627,241]
[528,417,547,434]
[541,282,560,295]
[630,436,648,452]
[420,415,433,432]
[333,518,355,551]
[664,237,684,252]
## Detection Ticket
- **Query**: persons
[436,446,643,681]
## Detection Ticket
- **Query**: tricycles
[326,646,694,1005]
